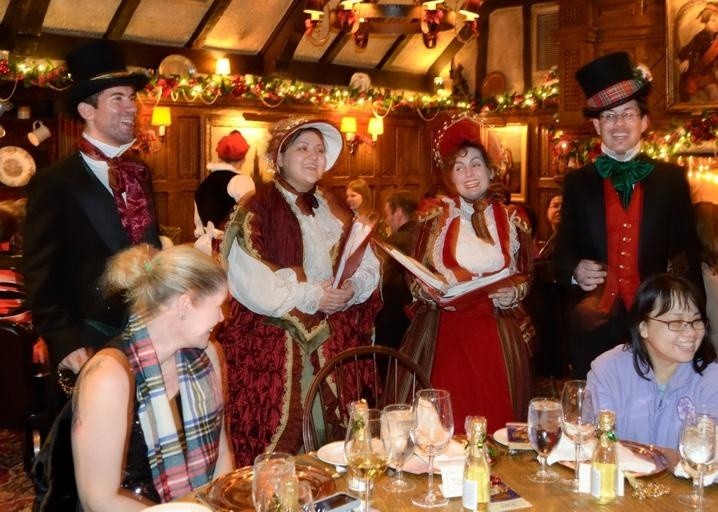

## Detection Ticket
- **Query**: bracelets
[513,283,523,304]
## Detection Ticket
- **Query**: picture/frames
[478,118,531,207]
[662,0,717,116]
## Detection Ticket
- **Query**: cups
[17,106,31,120]
[27,119,52,147]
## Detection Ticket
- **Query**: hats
[216,132,248,161]
[64,40,147,121]
[431,110,503,176]
[265,115,345,173]
[574,51,651,119]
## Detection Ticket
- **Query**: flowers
[634,62,653,85]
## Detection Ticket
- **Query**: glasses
[604,112,635,123]
[649,318,709,332]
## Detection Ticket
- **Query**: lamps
[215,57,232,88]
[340,114,359,154]
[148,101,174,141]
[365,115,385,145]
[299,0,481,52]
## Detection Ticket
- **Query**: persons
[579,272,716,464]
[499,143,514,185]
[69,241,236,510]
[555,82,708,380]
[382,140,534,436]
[19,75,161,376]
[346,179,381,225]
[378,190,423,362]
[534,191,562,255]
[216,116,381,473]
[694,202,717,348]
[192,133,254,238]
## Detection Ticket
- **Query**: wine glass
[527,399,563,484]
[344,399,386,511]
[410,386,453,509]
[381,402,416,494]
[560,377,598,490]
[676,412,718,512]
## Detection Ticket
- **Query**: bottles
[590,408,617,504]
[251,449,298,512]
[462,417,490,511]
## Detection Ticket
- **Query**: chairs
[32,392,82,512]
[302,341,435,461]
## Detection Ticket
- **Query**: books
[371,233,529,313]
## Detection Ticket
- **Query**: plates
[480,71,506,101]
[559,438,667,477]
[0,146,37,188]
[493,425,535,451]
[316,438,385,466]
[159,54,195,76]
[349,72,371,93]
[197,463,335,511]
